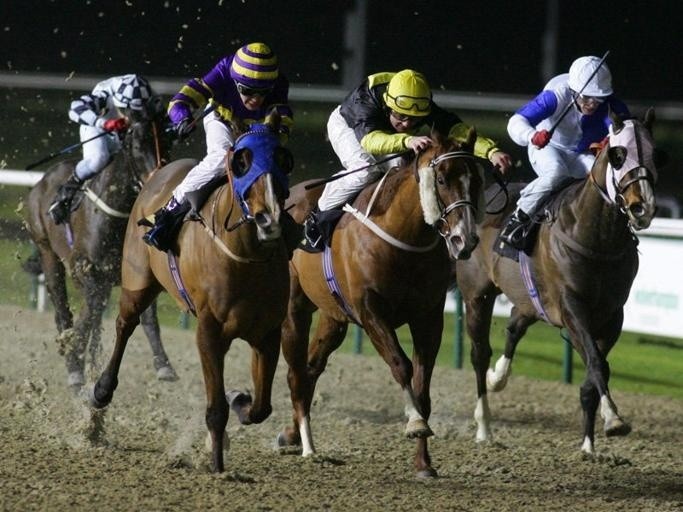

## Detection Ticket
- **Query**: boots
[300,203,327,253]
[142,205,175,253]
[47,171,83,225]
[497,205,527,251]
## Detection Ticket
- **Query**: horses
[89,107,290,474]
[277,121,482,481]
[446,102,658,456]
[21,102,180,391]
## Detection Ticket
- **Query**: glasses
[232,76,274,96]
[386,92,431,112]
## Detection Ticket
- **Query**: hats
[568,54,613,98]
[109,71,152,112]
[228,41,279,87]
[382,69,432,118]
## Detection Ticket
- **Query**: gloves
[172,117,195,142]
[529,129,551,148]
[101,116,129,134]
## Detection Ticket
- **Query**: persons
[141,41,297,252]
[46,73,172,225]
[498,55,632,248]
[300,69,517,250]
